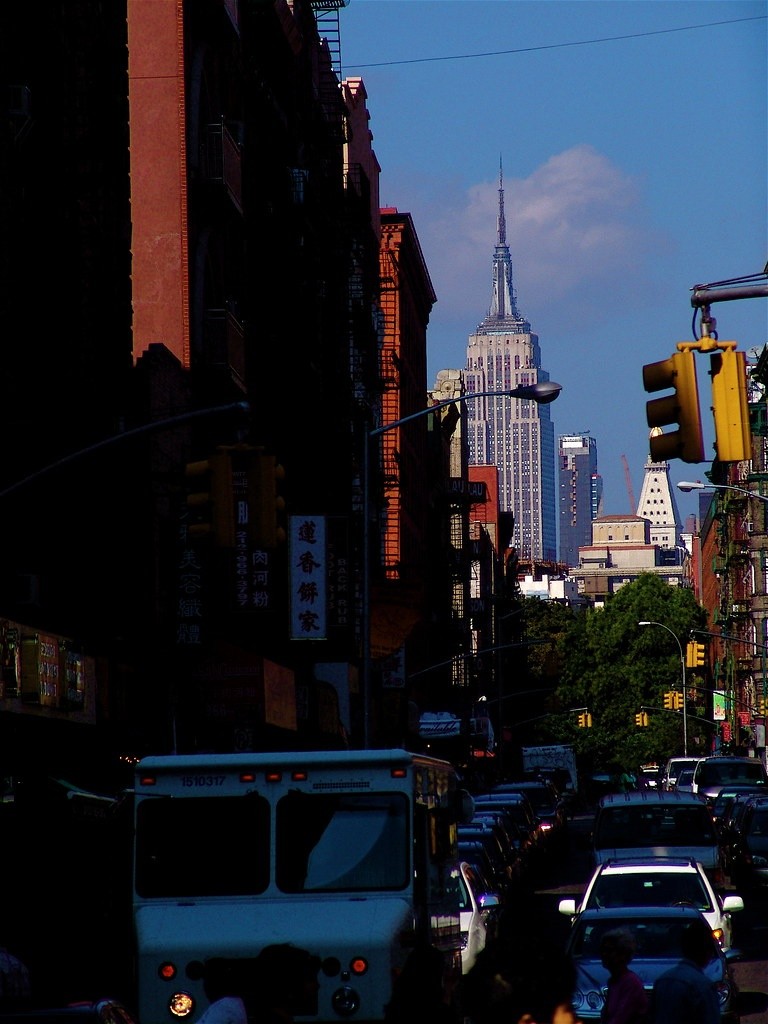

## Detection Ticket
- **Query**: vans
[590,789,731,898]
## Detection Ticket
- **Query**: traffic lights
[636,714,643,727]
[675,690,684,709]
[693,641,704,667]
[643,352,704,463]
[578,714,586,727]
[663,691,673,710]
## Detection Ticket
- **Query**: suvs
[556,853,745,963]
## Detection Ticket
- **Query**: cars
[0,990,139,1024]
[641,754,768,898]
[448,765,576,997]
[557,906,746,1024]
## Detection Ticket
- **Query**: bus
[131,742,476,1024]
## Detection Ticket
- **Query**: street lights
[499,597,569,722]
[639,621,688,758]
[361,380,564,750]
